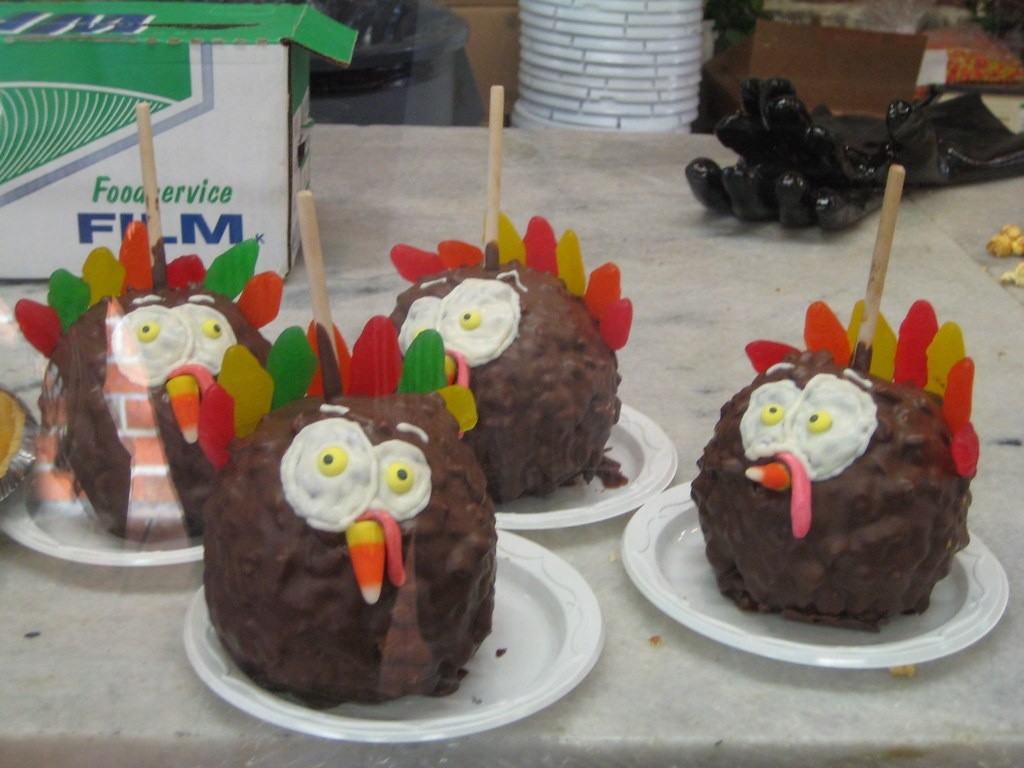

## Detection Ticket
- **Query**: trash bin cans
[306,2,486,125]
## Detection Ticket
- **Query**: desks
[0,123,1022,768]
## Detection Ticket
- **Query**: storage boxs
[0,0,359,282]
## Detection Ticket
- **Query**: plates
[492,402,679,532]
[0,475,206,567]
[620,479,1010,672]
[183,531,605,746]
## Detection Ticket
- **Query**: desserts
[9,101,286,550]
[376,83,636,506]
[193,185,501,713]
[689,162,981,637]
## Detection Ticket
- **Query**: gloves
[686,155,884,233]
[717,77,1024,186]
